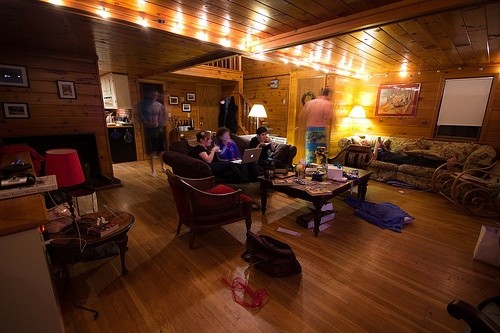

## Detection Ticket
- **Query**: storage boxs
[327,166,344,179]
[297,202,336,229]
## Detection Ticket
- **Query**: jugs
[264,158,275,179]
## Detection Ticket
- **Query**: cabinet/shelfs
[102,74,130,109]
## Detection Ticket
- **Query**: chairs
[165,170,254,248]
[432,159,500,212]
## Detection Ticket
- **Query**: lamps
[45,148,85,224]
[249,104,266,131]
[348,105,367,120]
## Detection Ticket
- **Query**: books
[306,185,332,196]
[272,179,292,185]
[79,217,119,239]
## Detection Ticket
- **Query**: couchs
[336,135,494,189]
[162,135,297,197]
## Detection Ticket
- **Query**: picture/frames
[170,97,179,105]
[182,103,191,111]
[0,64,30,86]
[2,102,29,118]
[186,92,195,101]
[375,83,421,117]
[57,80,77,99]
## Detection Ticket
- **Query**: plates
[307,172,325,178]
[262,143,270,146]
[210,150,221,154]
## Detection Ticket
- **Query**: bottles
[173,115,195,131]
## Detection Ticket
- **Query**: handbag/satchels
[474,225,500,268]
[241,231,303,278]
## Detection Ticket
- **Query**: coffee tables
[257,163,372,236]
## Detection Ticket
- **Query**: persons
[138,88,168,177]
[212,127,239,160]
[248,126,286,172]
[192,130,254,191]
[299,86,339,164]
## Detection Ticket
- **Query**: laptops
[230,148,262,164]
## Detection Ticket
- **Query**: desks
[54,210,135,288]
